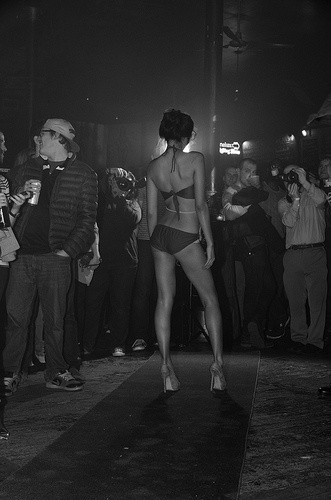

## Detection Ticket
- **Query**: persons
[79,166,144,357]
[308,158,331,348]
[1,131,32,378]
[145,109,230,393]
[13,129,102,375]
[276,164,328,354]
[222,158,288,351]
[126,166,155,351]
[1,118,98,397]
[206,164,238,225]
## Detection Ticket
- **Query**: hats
[41,118,76,144]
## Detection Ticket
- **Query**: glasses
[39,130,56,137]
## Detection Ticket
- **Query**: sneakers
[45,370,82,391]
[4,374,22,397]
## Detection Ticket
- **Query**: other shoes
[18,375,28,388]
[0,424,10,442]
[111,345,125,356]
[131,338,147,351]
[83,348,92,354]
[68,367,86,384]
[265,313,290,339]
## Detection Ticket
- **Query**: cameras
[281,169,299,185]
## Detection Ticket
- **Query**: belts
[292,243,324,250]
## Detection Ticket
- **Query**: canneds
[28,181,41,205]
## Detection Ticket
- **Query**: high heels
[160,364,181,393]
[210,360,226,391]
